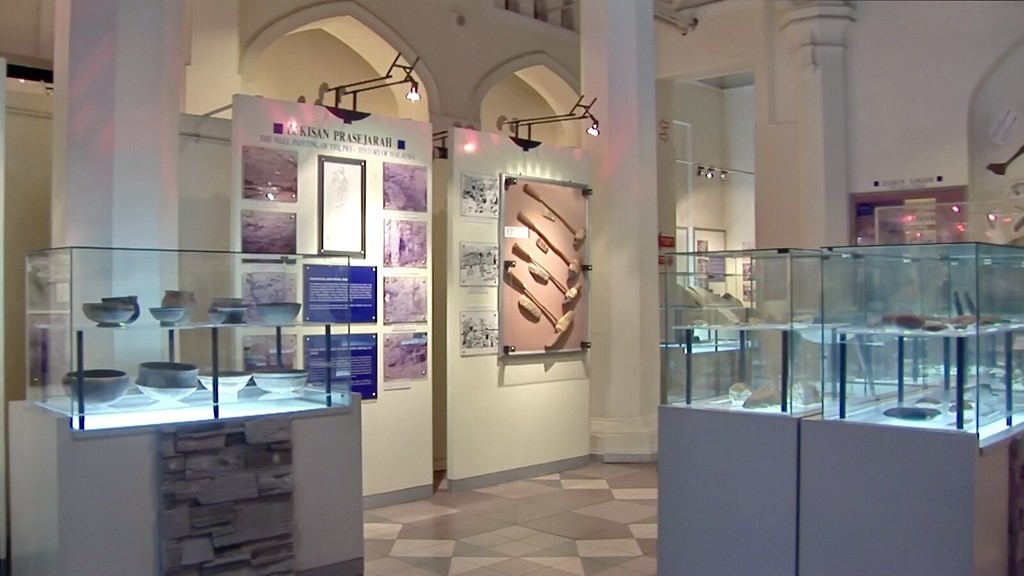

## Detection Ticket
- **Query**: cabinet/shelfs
[6,243,364,576]
[657,242,1024,576]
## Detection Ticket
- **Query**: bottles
[160,290,196,327]
[208,298,243,312]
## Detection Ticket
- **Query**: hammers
[524,183,586,246]
[518,212,583,278]
[512,243,581,306]
[506,266,577,348]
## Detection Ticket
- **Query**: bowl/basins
[195,371,253,401]
[149,307,187,327]
[81,302,135,328]
[214,307,249,323]
[101,295,140,324]
[208,311,227,324]
[62,369,130,408]
[135,361,199,409]
[255,302,301,325]
[252,369,310,400]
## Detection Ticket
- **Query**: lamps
[402,67,423,103]
[582,106,601,137]
[704,169,714,179]
